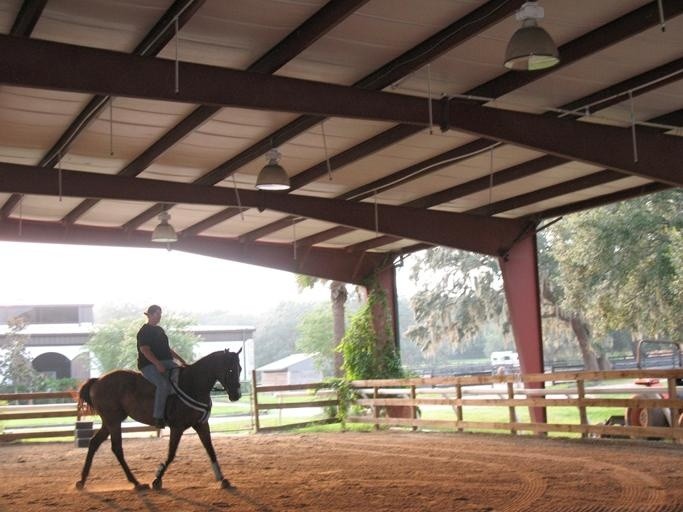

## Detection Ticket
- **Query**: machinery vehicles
[601,339,683,440]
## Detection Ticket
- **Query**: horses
[67,347,242,491]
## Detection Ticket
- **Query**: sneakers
[155,419,165,428]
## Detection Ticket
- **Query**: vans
[490,351,520,368]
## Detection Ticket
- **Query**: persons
[137,305,188,429]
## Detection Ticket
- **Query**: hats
[144,305,159,315]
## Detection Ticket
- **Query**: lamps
[256,148,292,192]
[150,212,179,245]
[502,1,562,72]
